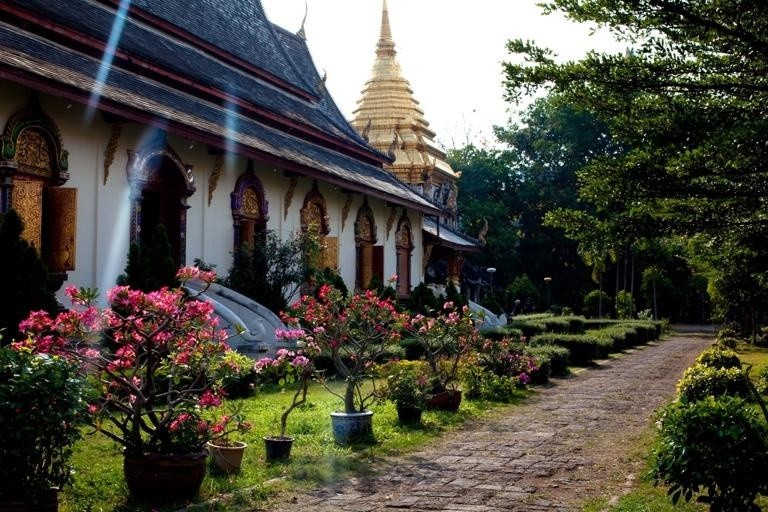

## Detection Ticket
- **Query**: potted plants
[1,344,90,512]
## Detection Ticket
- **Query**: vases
[207,439,247,474]
[263,436,295,463]
[122,451,206,505]
[398,408,421,428]
[332,408,373,444]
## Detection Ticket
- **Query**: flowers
[276,283,535,414]
[10,265,319,459]
